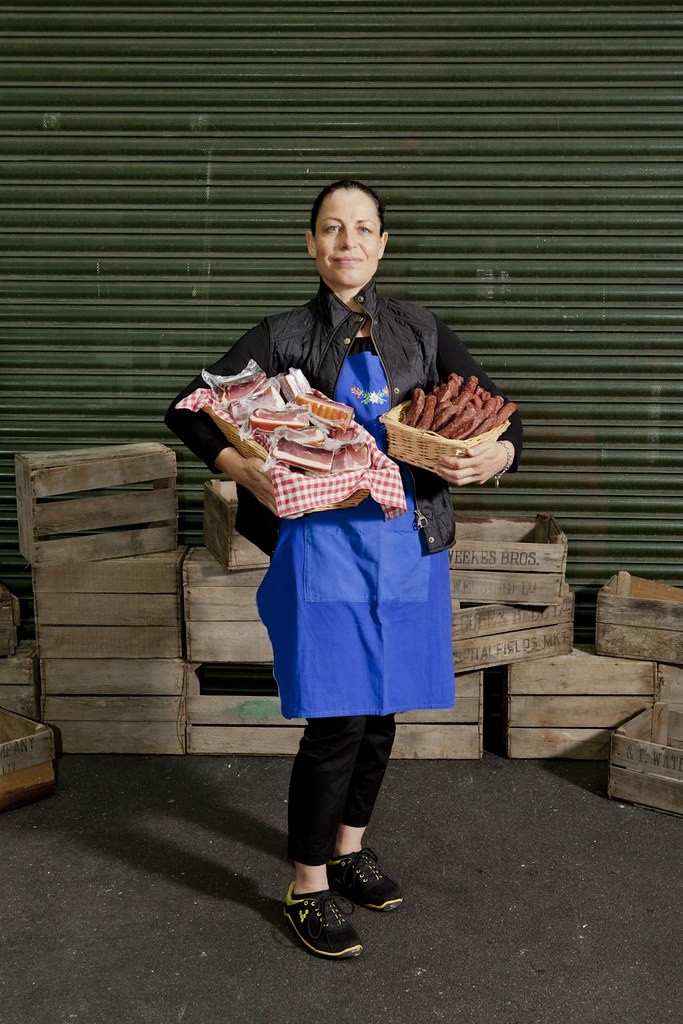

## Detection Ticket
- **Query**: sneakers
[283,880,364,959]
[326,847,404,911]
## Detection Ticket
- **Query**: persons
[162,179,525,960]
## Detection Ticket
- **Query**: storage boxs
[0,439,682,819]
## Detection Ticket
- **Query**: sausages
[403,372,517,440]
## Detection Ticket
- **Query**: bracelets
[494,442,512,488]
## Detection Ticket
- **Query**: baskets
[202,406,399,520]
[378,400,512,473]
